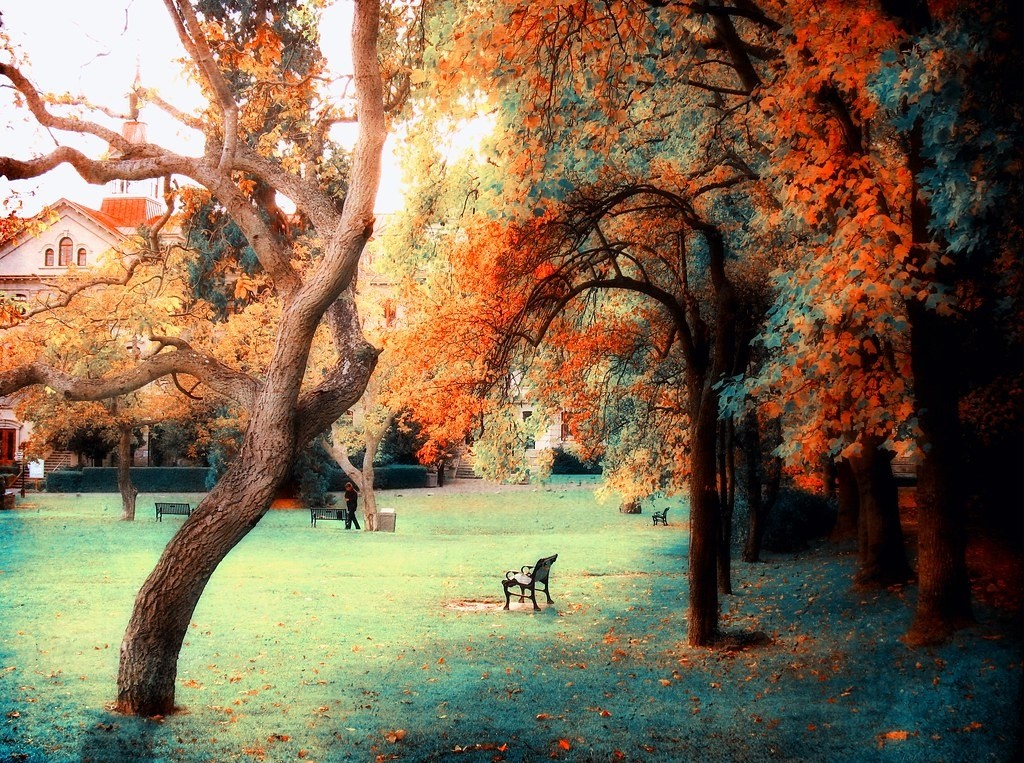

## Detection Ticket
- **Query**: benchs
[155,502,195,522]
[502,553,559,612]
[310,507,349,529]
[652,506,670,526]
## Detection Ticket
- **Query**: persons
[344,482,360,529]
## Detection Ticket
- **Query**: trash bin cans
[379,508,397,533]
[425,473,438,487]
[448,466,458,479]
